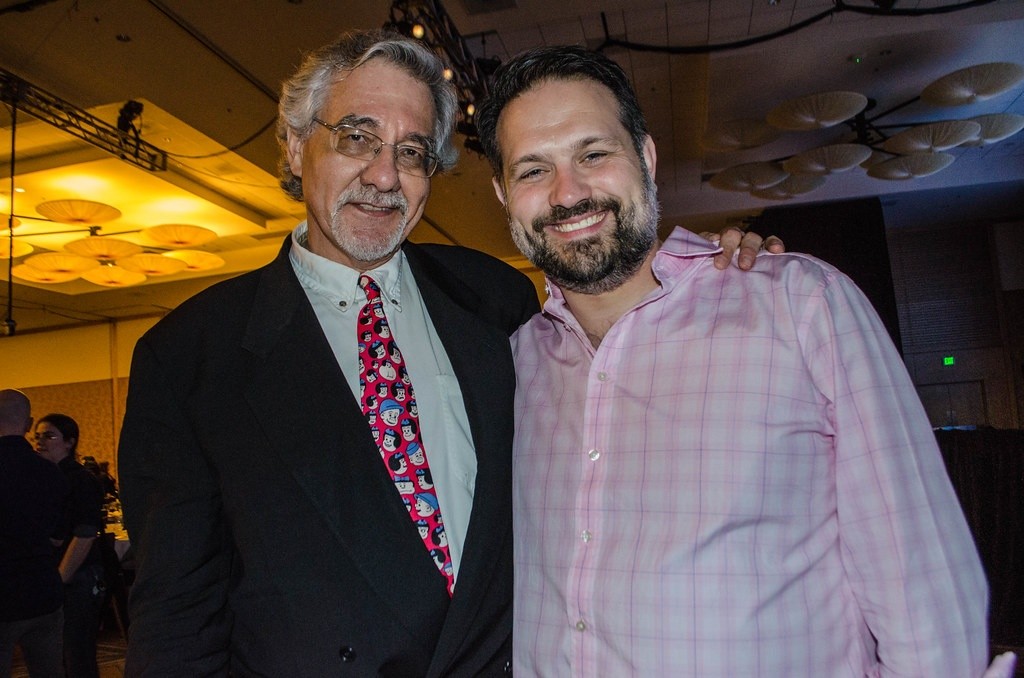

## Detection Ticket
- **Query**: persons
[0,389,66,678]
[473,44,1018,678]
[34,414,104,678]
[118,29,785,678]
[82,455,115,494]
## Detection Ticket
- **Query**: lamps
[698,62,1024,201]
[0,199,225,289]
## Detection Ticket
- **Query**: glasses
[312,117,441,180]
[30,432,60,442]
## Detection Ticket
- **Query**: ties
[357,276,454,598]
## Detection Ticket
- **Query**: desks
[104,522,131,646]
